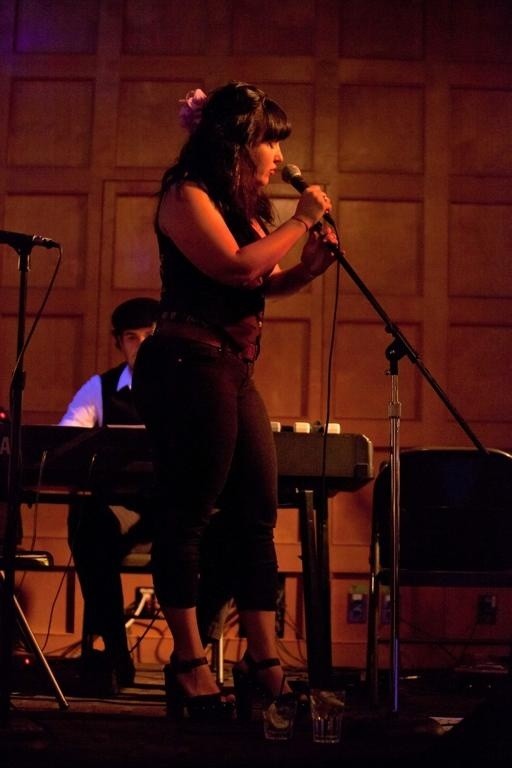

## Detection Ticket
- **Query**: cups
[261,690,299,741]
[311,686,343,745]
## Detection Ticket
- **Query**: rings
[323,196,328,202]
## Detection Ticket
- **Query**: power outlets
[474,592,498,626]
[380,590,403,625]
[133,586,157,619]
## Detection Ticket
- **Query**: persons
[130,80,348,726]
[54,297,161,687]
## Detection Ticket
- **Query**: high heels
[163,650,240,719]
[231,650,312,709]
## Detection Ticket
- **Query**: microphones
[280,162,335,229]
[1,227,56,254]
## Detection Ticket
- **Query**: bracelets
[290,216,309,233]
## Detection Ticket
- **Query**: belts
[155,318,258,363]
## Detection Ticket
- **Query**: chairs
[368,445,510,714]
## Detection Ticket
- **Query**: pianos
[0,421,374,506]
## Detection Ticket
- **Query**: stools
[80,551,226,691]
[1,548,72,712]
